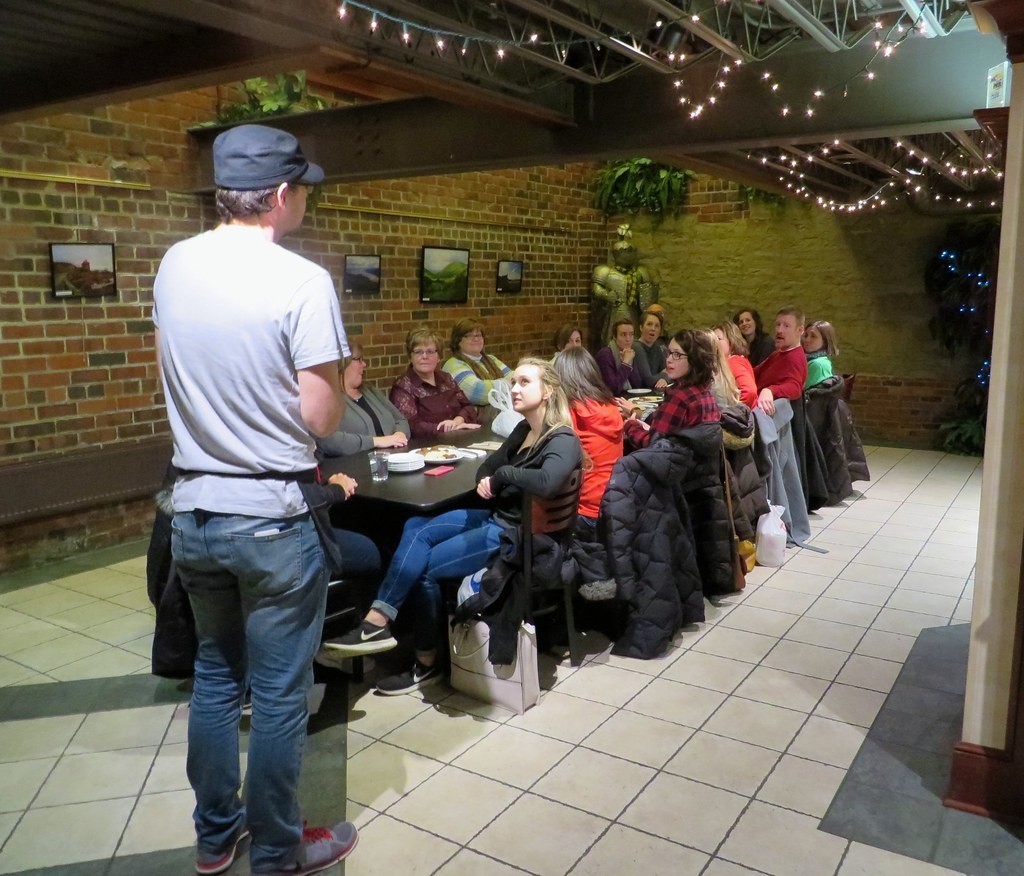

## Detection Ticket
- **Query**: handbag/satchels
[447,612,540,715]
[756,500,788,567]
[733,543,746,591]
[488,381,526,438]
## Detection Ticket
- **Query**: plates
[387,452,426,473]
[628,396,663,402]
[409,447,465,464]
[627,389,652,394]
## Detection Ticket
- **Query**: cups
[367,451,390,481]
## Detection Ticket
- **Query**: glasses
[463,334,483,339]
[664,350,686,360]
[411,349,437,355]
[350,355,364,363]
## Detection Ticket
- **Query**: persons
[321,305,840,695]
[150,124,359,876]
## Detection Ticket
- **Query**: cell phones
[423,465,454,476]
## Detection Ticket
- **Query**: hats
[213,124,324,188]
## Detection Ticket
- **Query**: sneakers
[265,820,359,876]
[321,622,398,658]
[376,659,443,695]
[195,804,250,873]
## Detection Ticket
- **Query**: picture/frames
[48,242,116,299]
[418,246,473,303]
[342,255,382,294]
[496,259,523,293]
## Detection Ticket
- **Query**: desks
[303,388,674,690]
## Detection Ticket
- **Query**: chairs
[136,370,870,716]
